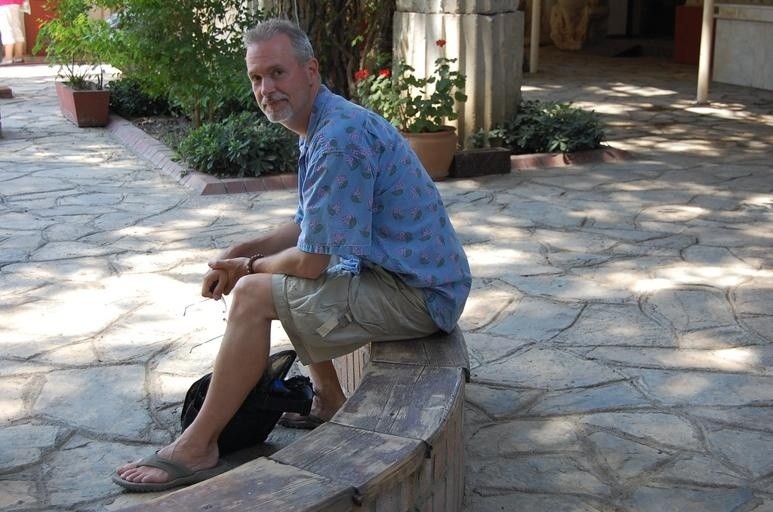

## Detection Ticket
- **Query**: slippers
[109,449,230,494]
[277,410,327,430]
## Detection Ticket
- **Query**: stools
[112,262,469,512]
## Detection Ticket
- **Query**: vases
[399,129,458,182]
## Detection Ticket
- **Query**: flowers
[352,38,468,135]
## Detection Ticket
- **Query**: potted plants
[31,2,120,128]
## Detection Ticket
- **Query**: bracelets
[243,251,264,273]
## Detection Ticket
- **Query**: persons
[112,17,470,490]
[0,1,31,67]
